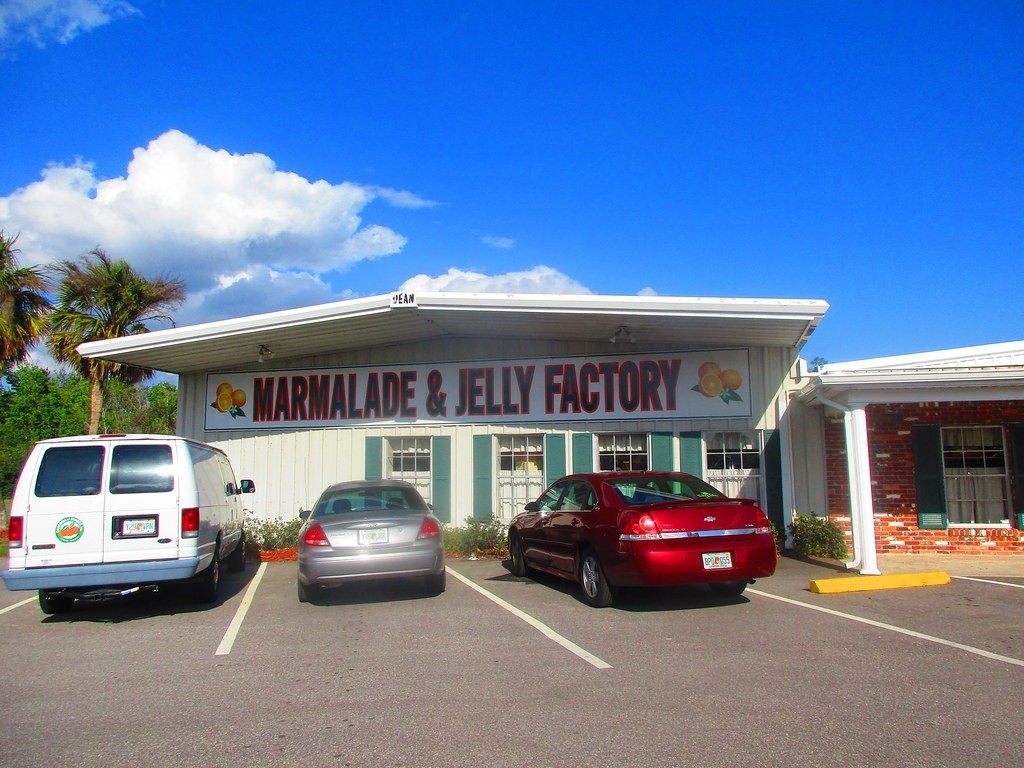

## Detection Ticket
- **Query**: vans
[0,435,255,614]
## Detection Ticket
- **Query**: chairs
[385,497,403,509]
[632,487,657,503]
[333,498,351,513]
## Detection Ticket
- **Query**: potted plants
[243,507,308,561]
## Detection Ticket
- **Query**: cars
[298,480,446,603]
[508,470,777,609]
